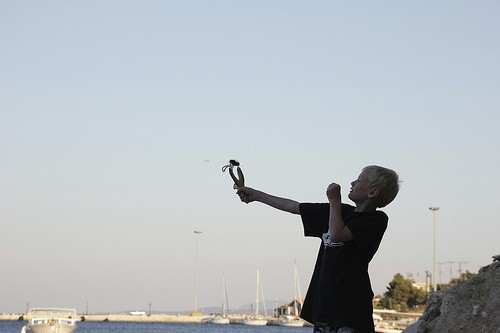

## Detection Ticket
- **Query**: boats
[242,269,268,326]
[269,259,304,326]
[20,308,82,333]
[208,276,230,324]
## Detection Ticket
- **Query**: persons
[236,165,403,333]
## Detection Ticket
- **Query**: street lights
[194,229,202,314]
[428,205,440,290]
